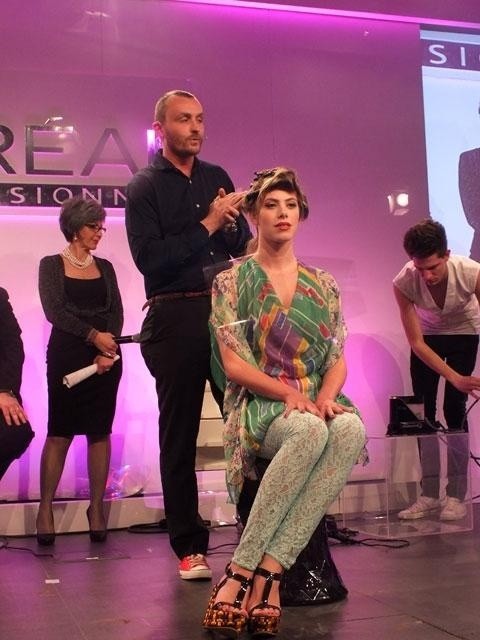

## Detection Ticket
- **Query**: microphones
[112,333,140,344]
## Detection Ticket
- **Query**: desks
[346,428,476,539]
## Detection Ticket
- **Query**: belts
[141,287,213,312]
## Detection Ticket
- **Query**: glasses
[84,218,107,235]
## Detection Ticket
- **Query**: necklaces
[61,246,96,270]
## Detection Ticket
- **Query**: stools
[232,470,349,609]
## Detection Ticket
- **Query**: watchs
[230,222,239,236]
[8,387,18,396]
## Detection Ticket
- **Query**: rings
[102,366,110,371]
[101,349,111,356]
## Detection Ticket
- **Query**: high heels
[36,512,56,546]
[85,504,108,543]
[201,560,250,640]
[246,565,284,640]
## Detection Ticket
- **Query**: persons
[391,218,480,524]
[0,286,36,549]
[35,194,123,548]
[124,88,255,581]
[199,166,369,639]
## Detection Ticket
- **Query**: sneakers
[439,496,468,522]
[397,494,442,520]
[177,550,213,583]
